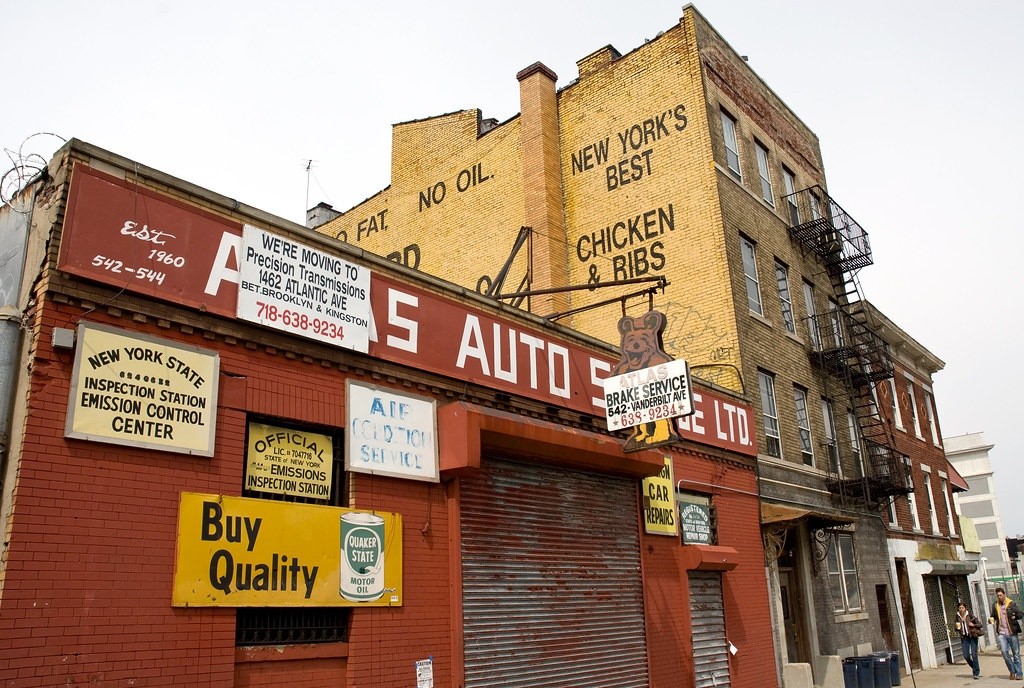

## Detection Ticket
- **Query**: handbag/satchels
[968,616,984,637]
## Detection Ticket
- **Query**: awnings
[760,502,859,532]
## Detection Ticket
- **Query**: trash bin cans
[847,656,875,688]
[867,652,893,688]
[889,651,901,686]
[842,659,860,688]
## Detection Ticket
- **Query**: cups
[989,617,995,625]
[956,621,961,630]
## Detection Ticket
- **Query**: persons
[953,602,985,680]
[988,588,1024,680]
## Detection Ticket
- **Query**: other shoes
[974,675,979,679]
[1016,675,1022,680]
[1010,673,1016,680]
